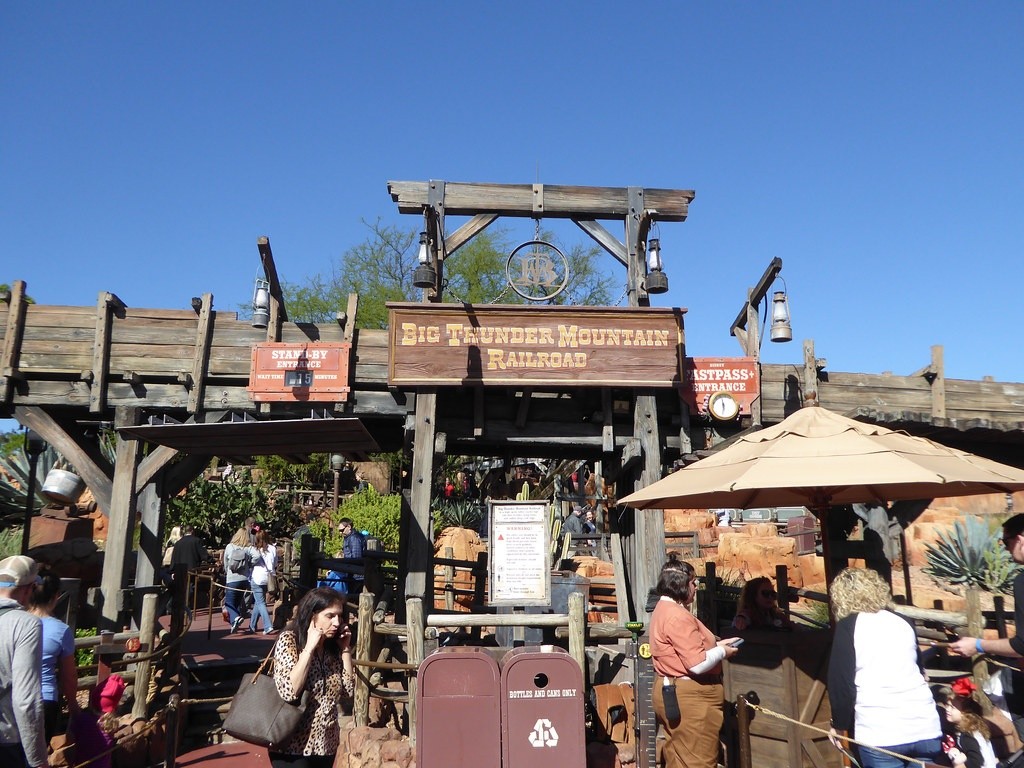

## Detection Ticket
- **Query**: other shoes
[230,616,244,634]
[263,628,273,635]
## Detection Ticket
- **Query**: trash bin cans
[413,648,501,768]
[502,645,587,768]
[550,569,591,626]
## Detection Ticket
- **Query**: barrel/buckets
[41,459,87,506]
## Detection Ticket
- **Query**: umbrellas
[614,391,1024,636]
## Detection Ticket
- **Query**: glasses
[339,526,347,532]
[1002,537,1011,546]
[758,589,776,599]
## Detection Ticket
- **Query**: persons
[0,516,367,768]
[440,465,596,572]
[648,512,1024,768]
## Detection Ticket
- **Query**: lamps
[414,207,435,288]
[645,221,669,293]
[769,274,793,341]
[252,253,271,328]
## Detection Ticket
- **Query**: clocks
[709,390,739,421]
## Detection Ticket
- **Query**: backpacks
[229,543,248,573]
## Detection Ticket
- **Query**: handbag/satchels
[267,572,280,600]
[221,629,308,754]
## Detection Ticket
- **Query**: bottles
[775,608,785,627]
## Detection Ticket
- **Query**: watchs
[976,637,985,654]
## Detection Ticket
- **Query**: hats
[573,506,586,511]
[0,556,38,587]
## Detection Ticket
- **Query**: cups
[540,644,554,653]
[765,604,777,624]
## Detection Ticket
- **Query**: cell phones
[730,638,745,647]
[337,623,344,639]
